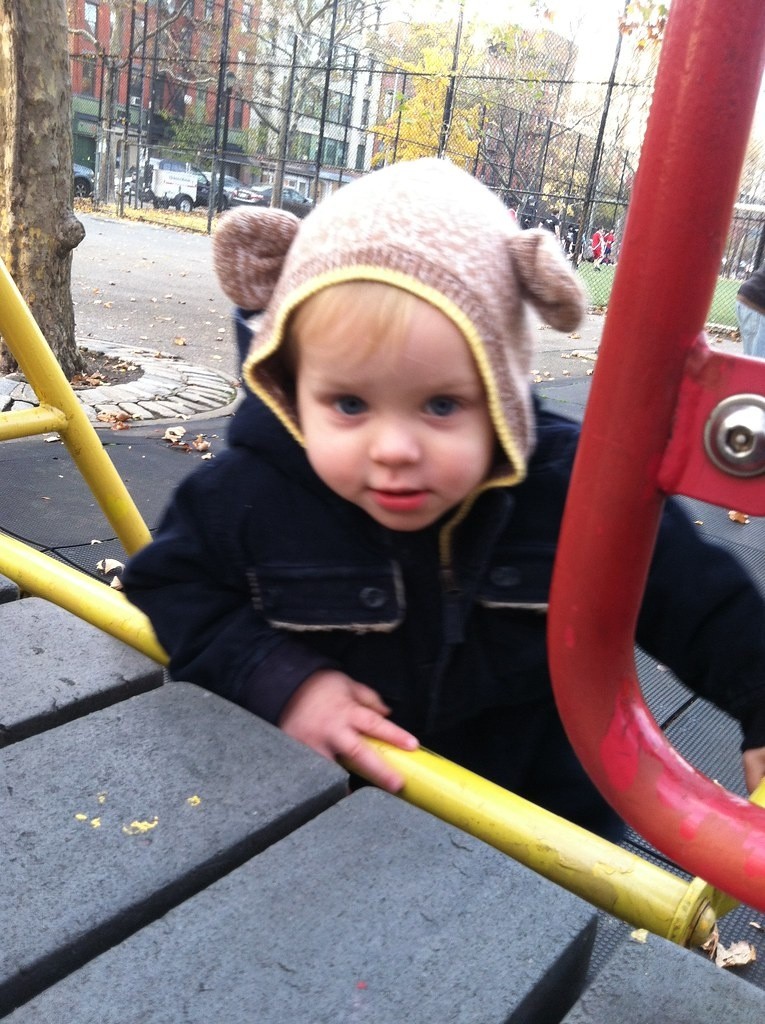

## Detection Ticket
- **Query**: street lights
[214,70,236,216]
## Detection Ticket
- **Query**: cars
[114,157,243,218]
[234,183,313,220]
[73,160,97,199]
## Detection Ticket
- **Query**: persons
[735,258,765,360]
[117,158,765,846]
[505,202,615,271]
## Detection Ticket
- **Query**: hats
[208,154,596,579]
